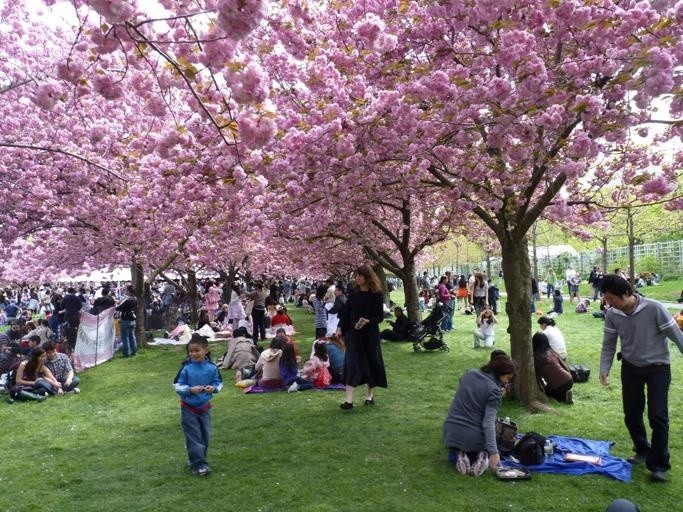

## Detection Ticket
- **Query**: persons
[442,354,530,476]
[147,275,345,393]
[173,336,221,477]
[0,281,138,399]
[529,330,579,406]
[490,349,505,357]
[336,263,384,409]
[598,275,682,481]
[530,262,666,325]
[537,315,568,361]
[385,271,500,348]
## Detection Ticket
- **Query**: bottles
[236,371,242,382]
[502,416,511,425]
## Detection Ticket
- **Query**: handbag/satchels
[238,366,258,381]
[515,432,547,465]
[494,418,517,460]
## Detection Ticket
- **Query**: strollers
[407,302,453,354]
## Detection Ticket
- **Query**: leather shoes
[340,402,353,410]
[650,470,669,481]
[364,398,374,405]
[628,453,645,464]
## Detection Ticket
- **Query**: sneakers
[164,331,172,338]
[288,382,299,394]
[455,450,470,475]
[563,390,574,404]
[7,387,80,407]
[199,463,208,475]
[190,465,213,475]
[470,450,490,477]
